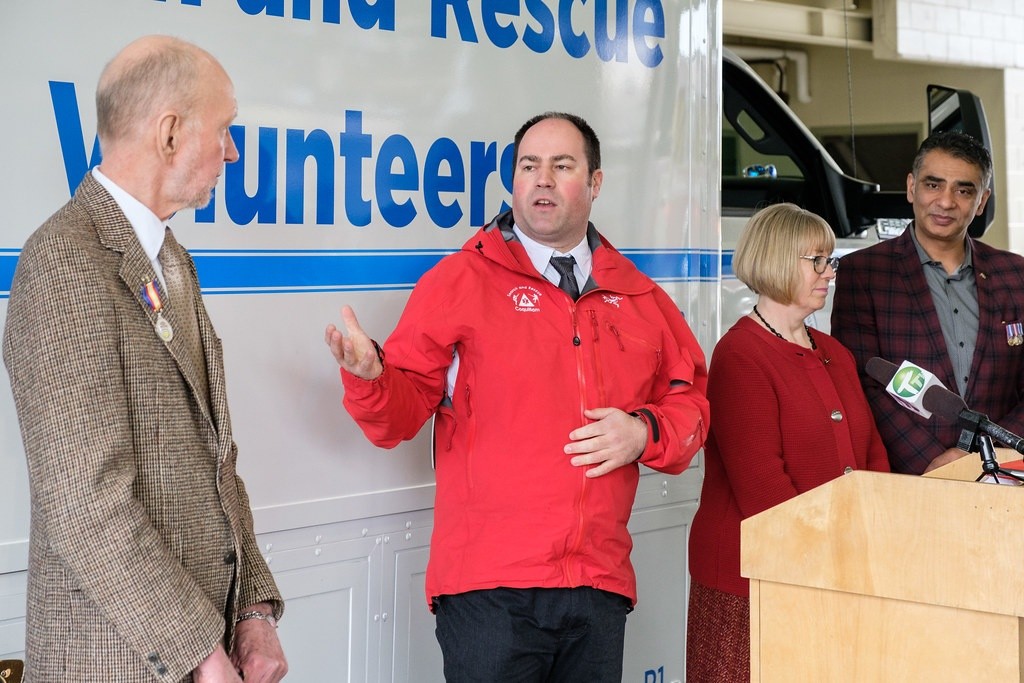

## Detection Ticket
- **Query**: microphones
[865,357,967,424]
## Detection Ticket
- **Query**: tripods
[975,435,1024,485]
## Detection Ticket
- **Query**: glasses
[799,255,840,274]
[743,163,778,178]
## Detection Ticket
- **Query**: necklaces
[753,305,816,350]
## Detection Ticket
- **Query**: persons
[2,36,294,683]
[687,202,891,683]
[830,130,1024,476]
[324,111,710,683]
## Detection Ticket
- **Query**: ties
[550,254,579,304]
[156,227,210,413]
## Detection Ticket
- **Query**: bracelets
[236,610,279,628]
